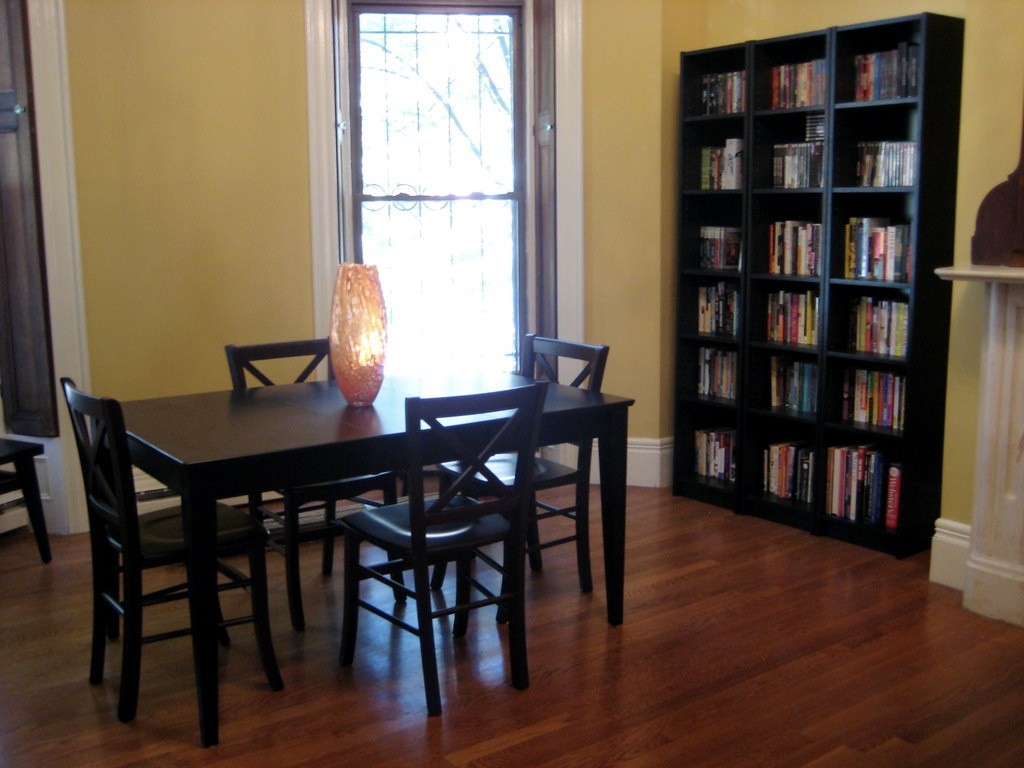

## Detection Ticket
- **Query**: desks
[110,365,635,748]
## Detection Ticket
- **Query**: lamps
[329,263,387,405]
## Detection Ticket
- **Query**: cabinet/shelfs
[676,13,965,561]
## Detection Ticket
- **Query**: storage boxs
[723,138,742,190]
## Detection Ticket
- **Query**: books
[702,68,746,116]
[844,216,911,284]
[700,138,743,190]
[768,219,821,277]
[824,444,901,529]
[694,428,736,483]
[846,294,908,358]
[770,355,819,414]
[697,287,739,337]
[697,347,736,400]
[700,226,743,271]
[853,42,920,102]
[842,369,905,431]
[854,141,916,187]
[773,115,825,189]
[763,440,814,504]
[770,58,826,109]
[768,289,819,346]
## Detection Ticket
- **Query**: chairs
[435,333,609,626]
[225,337,408,629]
[337,380,549,716]
[0,437,52,565]
[58,376,286,724]
